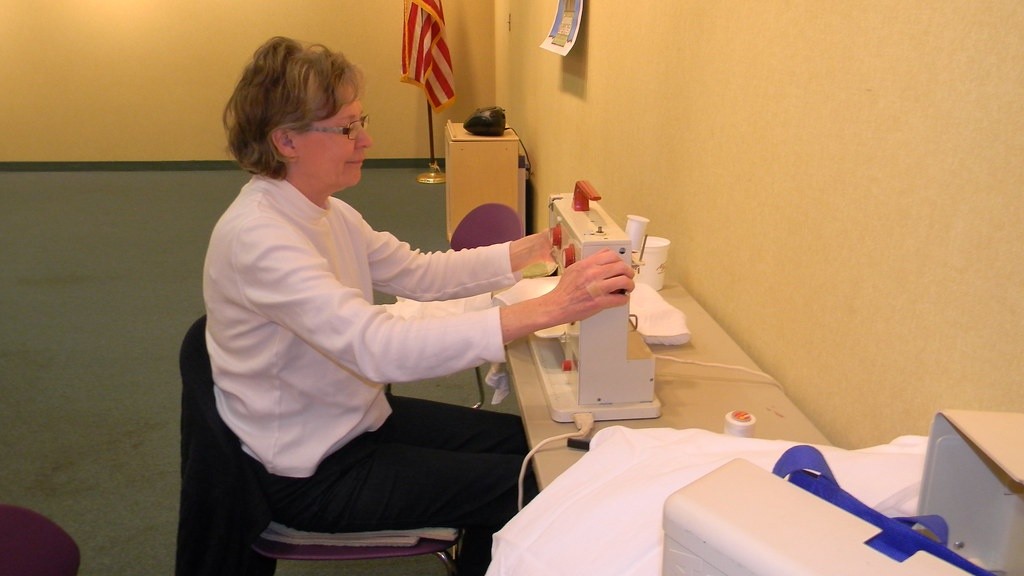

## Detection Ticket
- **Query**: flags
[402,0,456,112]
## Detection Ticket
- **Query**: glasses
[305,113,369,140]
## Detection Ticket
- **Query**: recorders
[463,106,506,136]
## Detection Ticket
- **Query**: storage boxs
[663,457,979,575]
[917,409,1024,576]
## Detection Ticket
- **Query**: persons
[202,37,634,576]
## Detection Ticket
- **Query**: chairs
[181,313,460,576]
[451,202,524,254]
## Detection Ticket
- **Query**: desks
[497,272,832,576]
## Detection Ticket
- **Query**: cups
[639,236,671,292]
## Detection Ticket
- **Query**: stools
[0,503,81,576]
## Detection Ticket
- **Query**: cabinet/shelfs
[443,119,531,255]
